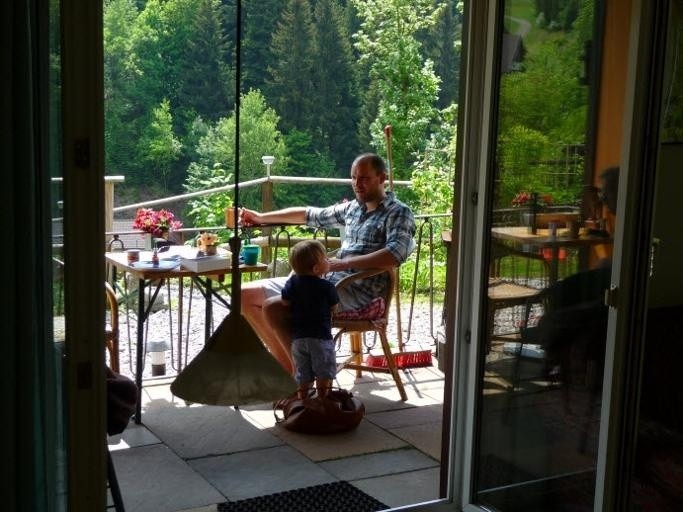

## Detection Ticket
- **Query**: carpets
[217,479,391,512]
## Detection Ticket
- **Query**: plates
[133,262,176,272]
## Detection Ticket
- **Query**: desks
[106,246,268,424]
[492,226,615,428]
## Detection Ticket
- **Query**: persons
[519,164,618,377]
[282,238,340,398]
[240,152,417,408]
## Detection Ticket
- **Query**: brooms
[368,125,433,369]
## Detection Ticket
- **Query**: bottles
[152,248,158,262]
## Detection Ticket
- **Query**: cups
[196,233,217,253]
[243,245,258,265]
[125,249,140,264]
[205,245,216,255]
[225,207,242,228]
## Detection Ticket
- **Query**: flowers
[511,191,554,208]
[132,207,182,237]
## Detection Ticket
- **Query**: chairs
[325,247,407,402]
[53,257,119,376]
[485,275,548,426]
